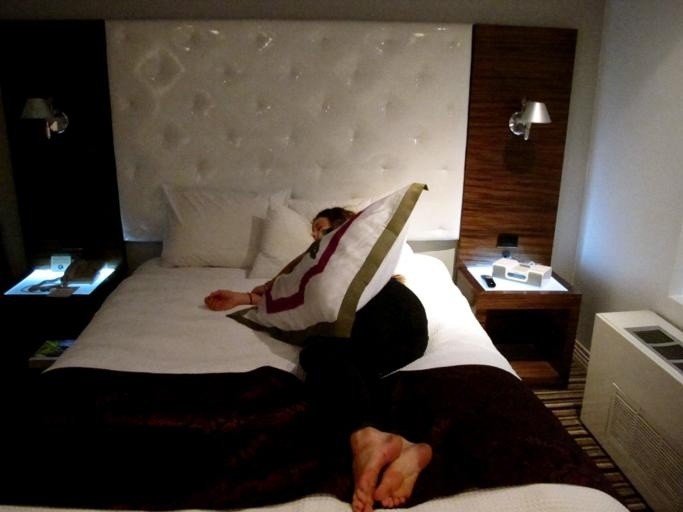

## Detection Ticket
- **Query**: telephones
[61,256,105,281]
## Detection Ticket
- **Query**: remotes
[481,274,496,287]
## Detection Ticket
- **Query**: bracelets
[247,292,254,304]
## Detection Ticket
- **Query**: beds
[1,14,634,511]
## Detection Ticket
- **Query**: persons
[205,206,433,512]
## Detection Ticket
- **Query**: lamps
[507,97,553,143]
[19,95,69,143]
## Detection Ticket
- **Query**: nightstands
[455,260,582,389]
[4,259,121,384]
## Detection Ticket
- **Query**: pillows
[153,183,295,269]
[246,193,316,281]
[223,179,428,346]
[283,192,377,220]
